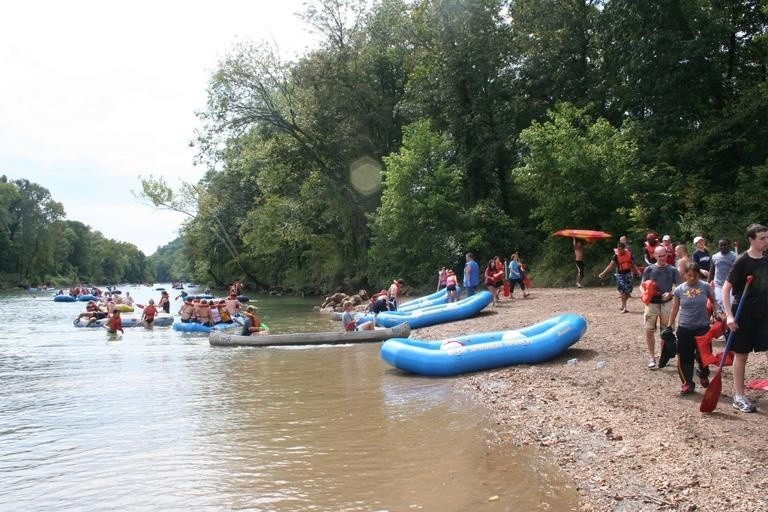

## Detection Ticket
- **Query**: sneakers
[732,396,756,413]
[680,382,695,395]
[648,358,655,367]
[699,378,709,388]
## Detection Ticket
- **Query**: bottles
[567,358,577,366]
[596,360,606,367]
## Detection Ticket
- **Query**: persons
[439,248,531,309]
[341,300,376,332]
[29,275,270,336]
[572,237,592,288]
[598,223,768,416]
[314,277,400,313]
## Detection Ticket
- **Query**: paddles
[503,257,509,296]
[699,274,753,413]
[516,250,530,289]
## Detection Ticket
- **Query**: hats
[693,236,704,244]
[662,235,670,240]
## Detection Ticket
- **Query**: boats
[173,315,244,333]
[78,293,97,301]
[157,288,165,291]
[376,291,494,329]
[188,285,195,288]
[229,295,250,302]
[350,285,463,326]
[176,286,183,290]
[208,320,412,347]
[552,229,613,241]
[382,315,587,377]
[73,312,174,329]
[31,285,54,292]
[54,295,75,302]
[183,294,213,301]
